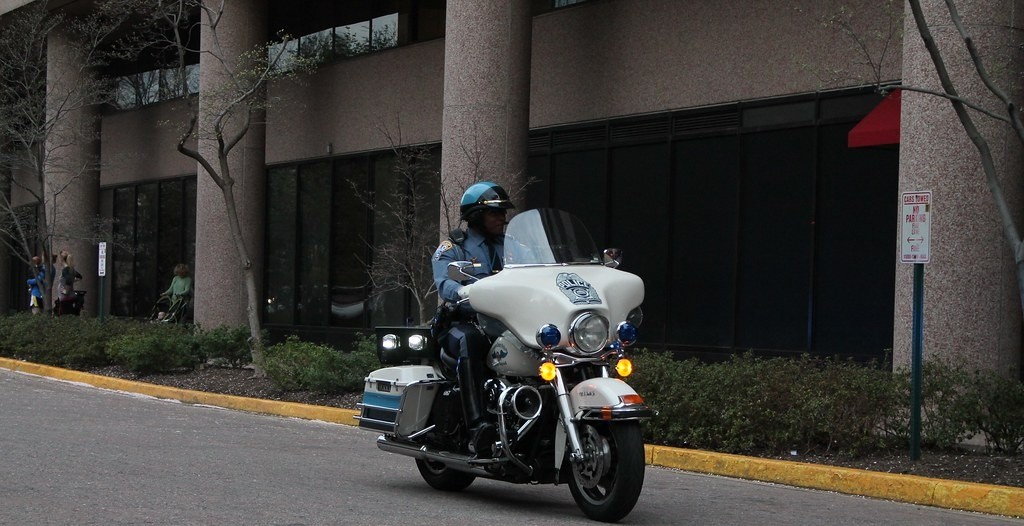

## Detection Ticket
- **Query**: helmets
[460,181,515,227]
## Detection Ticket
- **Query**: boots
[456,356,497,459]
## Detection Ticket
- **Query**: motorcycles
[352,208,653,521]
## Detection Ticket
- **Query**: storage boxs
[359,365,440,435]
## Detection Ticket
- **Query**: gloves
[457,284,472,298]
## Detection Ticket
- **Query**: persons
[156,264,192,324]
[27,251,57,315]
[431,182,539,461]
[57,250,83,317]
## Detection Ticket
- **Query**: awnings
[848,89,901,147]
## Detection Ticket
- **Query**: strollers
[144,295,187,325]
[53,290,87,316]
